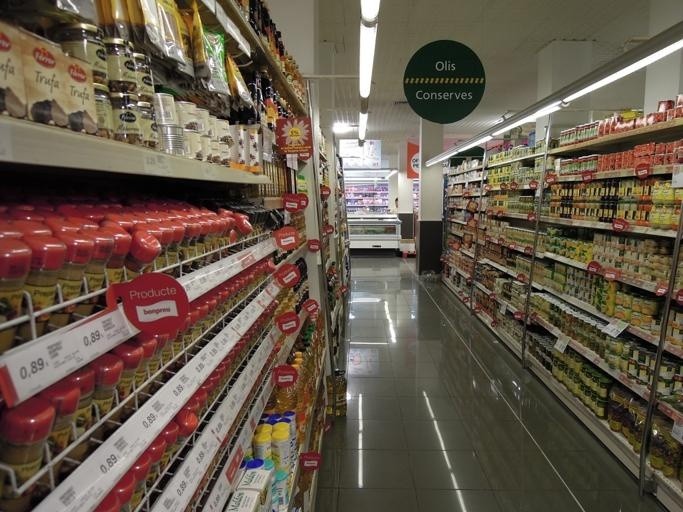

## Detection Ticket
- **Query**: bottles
[292,209,307,249]
[326,368,347,417]
[249,0,305,108]
[233,65,295,132]
[253,308,326,512]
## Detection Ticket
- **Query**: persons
[388,197,398,215]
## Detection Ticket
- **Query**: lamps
[425,20,683,168]
[358,0,381,145]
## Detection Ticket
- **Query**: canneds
[247,411,300,512]
[487,108,681,479]
[53,22,235,168]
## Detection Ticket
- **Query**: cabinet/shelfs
[440,117,683,512]
[346,190,389,207]
[0,0,344,512]
[347,213,401,249]
